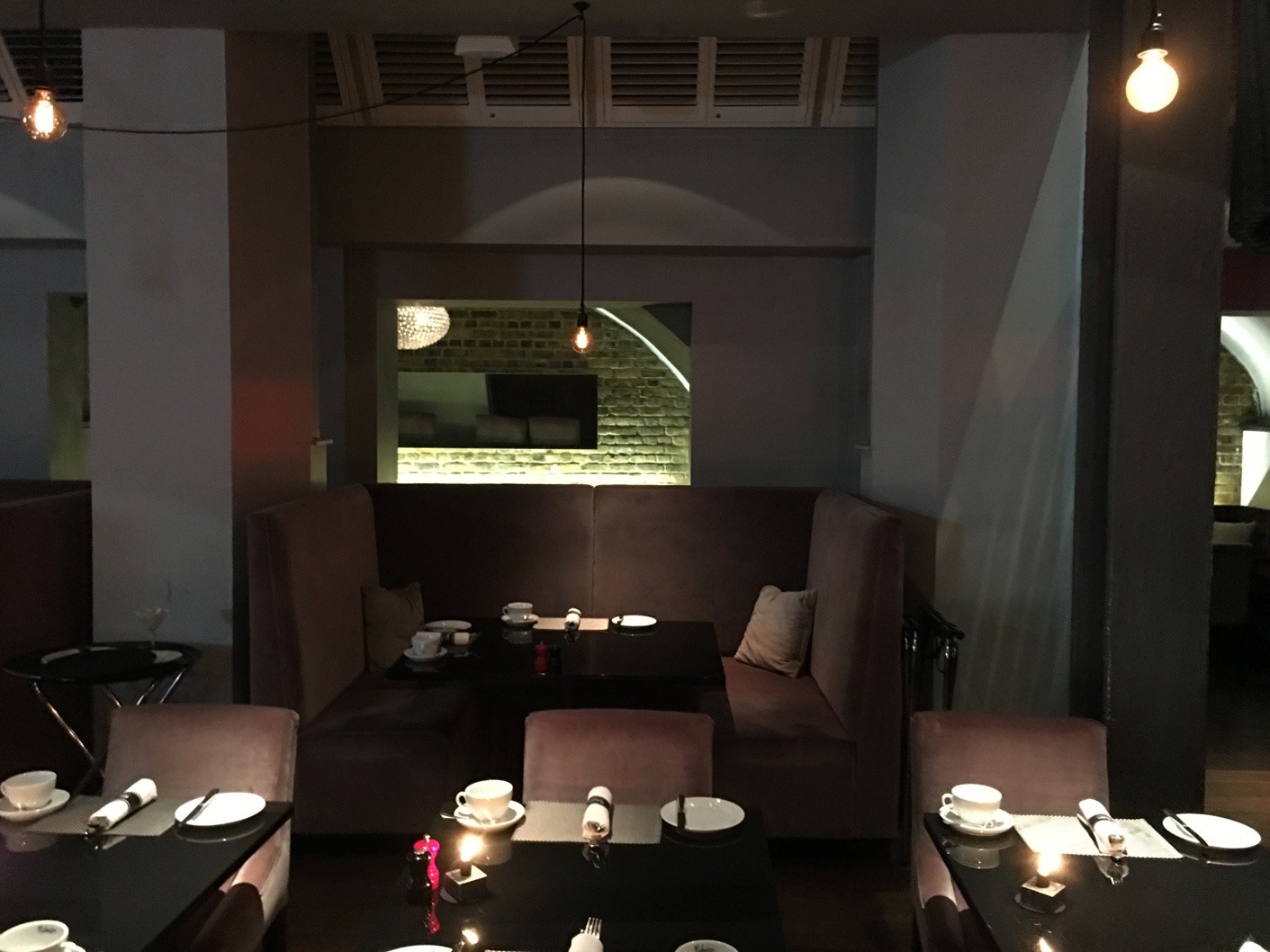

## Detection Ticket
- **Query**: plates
[175,792,266,827]
[404,646,448,661]
[425,620,472,632]
[0,789,71,821]
[660,796,745,831]
[675,940,738,952]
[388,945,453,952]
[939,803,1014,836]
[36,942,86,952]
[453,800,525,833]
[502,614,539,626]
[1162,813,1261,850]
[612,615,657,626]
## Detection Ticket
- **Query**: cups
[411,635,439,655]
[0,771,57,809]
[503,602,533,620]
[942,783,1003,824]
[456,779,513,820]
[0,920,70,952]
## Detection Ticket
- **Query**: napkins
[564,608,582,630]
[582,786,613,840]
[1077,799,1128,855]
[88,778,158,830]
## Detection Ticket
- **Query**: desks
[387,613,727,803]
[362,794,787,952]
[923,813,1270,952]
[0,639,294,952]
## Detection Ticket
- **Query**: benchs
[246,483,904,839]
[0,479,93,794]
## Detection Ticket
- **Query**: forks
[585,917,601,938]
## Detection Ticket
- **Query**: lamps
[455,35,515,58]
[397,307,450,350]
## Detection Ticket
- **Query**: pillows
[360,582,424,678]
[734,584,818,679]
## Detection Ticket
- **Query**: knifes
[676,793,687,834]
[178,788,220,827]
[615,613,624,628]
[1164,809,1209,848]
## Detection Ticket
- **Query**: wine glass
[132,579,172,647]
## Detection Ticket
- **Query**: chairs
[910,710,1110,952]
[522,709,716,804]
[398,412,440,447]
[1207,505,1270,700]
[102,703,299,952]
[476,413,581,448]
